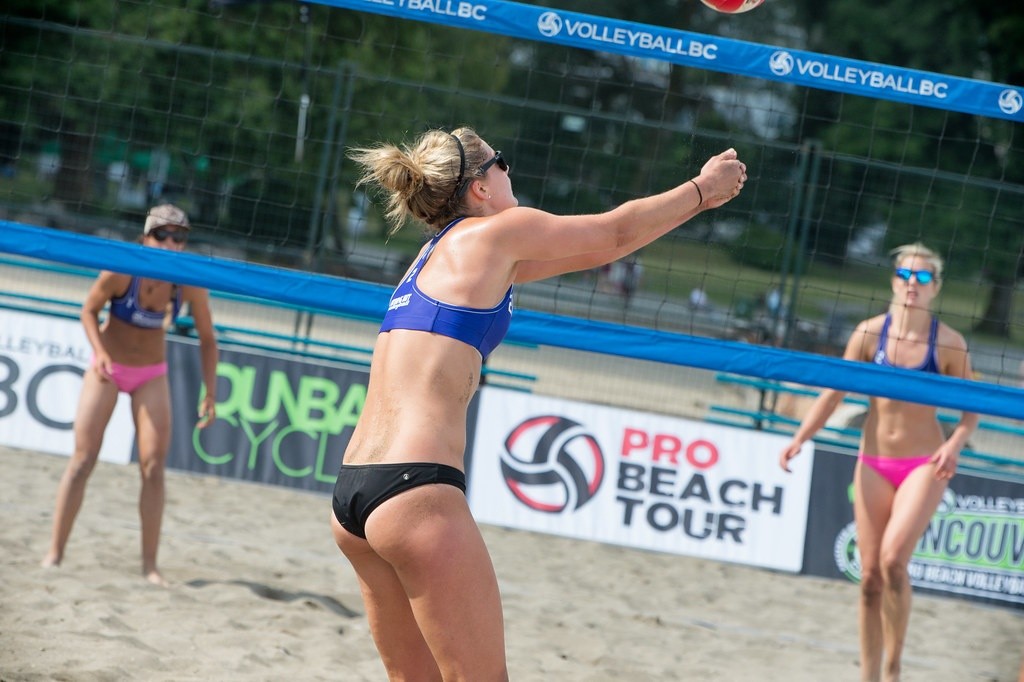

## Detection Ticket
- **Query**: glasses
[459,150,508,196]
[894,267,935,285]
[149,228,186,243]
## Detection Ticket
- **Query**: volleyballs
[699,0,765,14]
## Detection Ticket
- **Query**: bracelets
[689,180,702,207]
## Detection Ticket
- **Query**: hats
[144,205,190,236]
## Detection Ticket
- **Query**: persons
[328,126,751,682]
[0,126,872,353]
[42,203,218,585]
[780,241,981,682]
